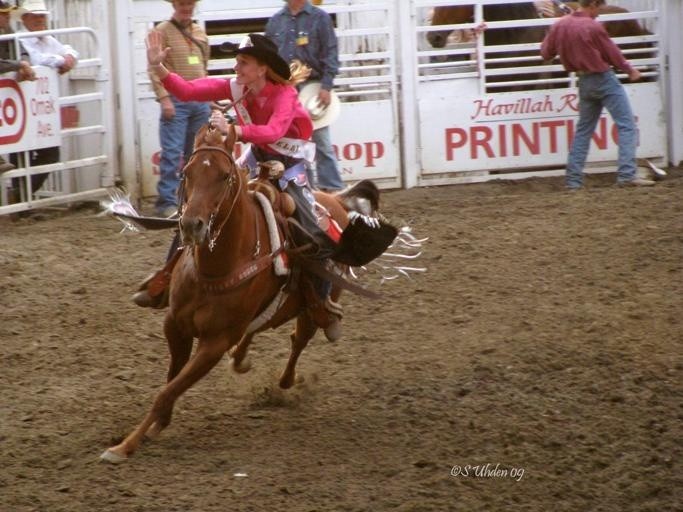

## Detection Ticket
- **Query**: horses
[100,122,380,466]
[425,3,658,175]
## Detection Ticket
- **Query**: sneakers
[616,176,655,190]
[324,309,342,343]
[131,289,162,307]
[161,204,179,219]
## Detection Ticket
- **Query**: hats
[219,32,292,80]
[0,0,21,14]
[15,0,51,22]
[298,82,341,131]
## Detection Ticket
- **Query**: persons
[148,1,212,215]
[7,0,79,221]
[0,0,35,170]
[129,29,342,341]
[540,0,656,190]
[264,1,345,193]
